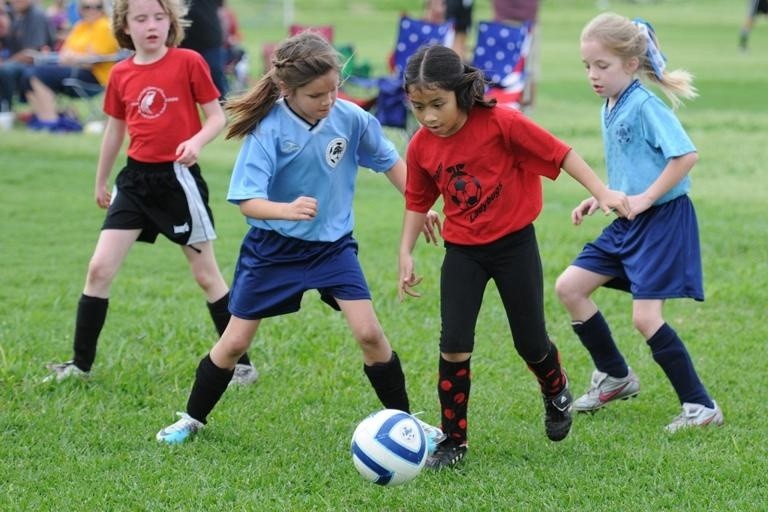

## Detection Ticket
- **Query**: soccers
[349,409,428,488]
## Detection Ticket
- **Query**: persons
[218,0,252,92]
[155,32,447,456]
[23,0,122,132]
[740,1,768,50]
[43,0,259,388]
[553,12,724,436]
[176,1,229,106]
[398,46,633,470]
[0,0,71,107]
[428,0,474,58]
[490,0,540,106]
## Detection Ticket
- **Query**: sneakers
[153,412,203,448]
[541,367,573,442]
[660,398,722,440]
[41,362,91,384]
[570,365,639,413]
[226,360,259,391]
[409,413,447,445]
[421,440,469,474]
